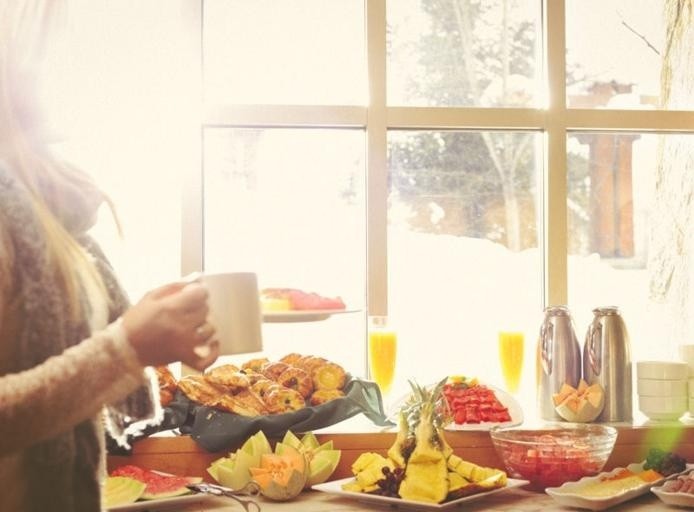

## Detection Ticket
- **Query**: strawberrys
[444,383,512,424]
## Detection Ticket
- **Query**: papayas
[551,378,605,422]
[205,428,343,503]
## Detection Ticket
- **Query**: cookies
[178,351,346,417]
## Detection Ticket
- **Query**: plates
[311,475,530,511]
[105,468,235,512]
[437,378,523,431]
[262,307,366,324]
[544,460,694,512]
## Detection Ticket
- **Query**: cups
[191,271,263,358]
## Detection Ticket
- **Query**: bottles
[536,304,633,423]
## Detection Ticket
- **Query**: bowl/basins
[491,422,618,489]
[636,360,694,423]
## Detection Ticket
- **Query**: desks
[92,424,692,511]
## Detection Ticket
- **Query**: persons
[1,1,220,511]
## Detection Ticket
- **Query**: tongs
[185,482,265,512]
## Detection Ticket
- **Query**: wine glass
[364,315,399,415]
[495,330,526,396]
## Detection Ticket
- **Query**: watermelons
[111,463,204,499]
